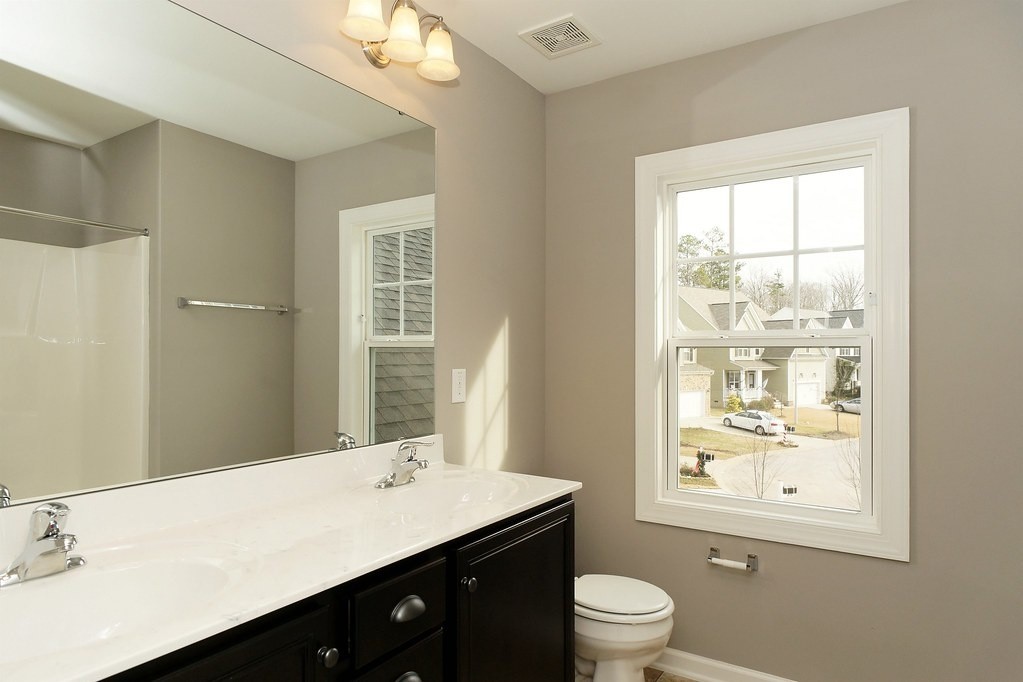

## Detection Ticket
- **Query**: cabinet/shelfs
[98,492,575,682]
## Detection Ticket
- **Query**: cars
[721,409,786,435]
[829,398,860,415]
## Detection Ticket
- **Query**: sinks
[346,470,531,532]
[1,540,269,661]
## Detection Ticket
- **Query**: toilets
[574,574,675,682]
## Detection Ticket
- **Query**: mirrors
[0,0,439,509]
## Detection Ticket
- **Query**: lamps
[341,0,461,82]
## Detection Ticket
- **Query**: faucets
[374,441,435,489]
[328,431,357,452]
[0,502,88,588]
